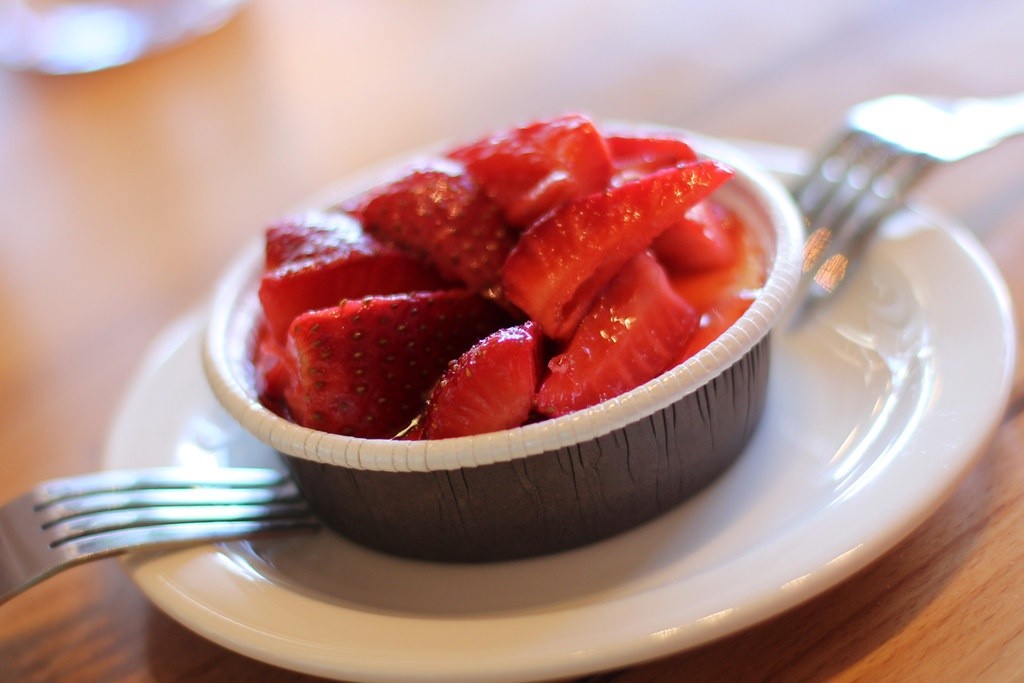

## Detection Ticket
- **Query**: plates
[101,118,1017,680]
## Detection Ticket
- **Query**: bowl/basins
[202,113,807,562]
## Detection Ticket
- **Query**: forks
[0,466,324,610]
[790,93,1022,338]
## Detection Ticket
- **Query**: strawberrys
[255,114,761,439]
